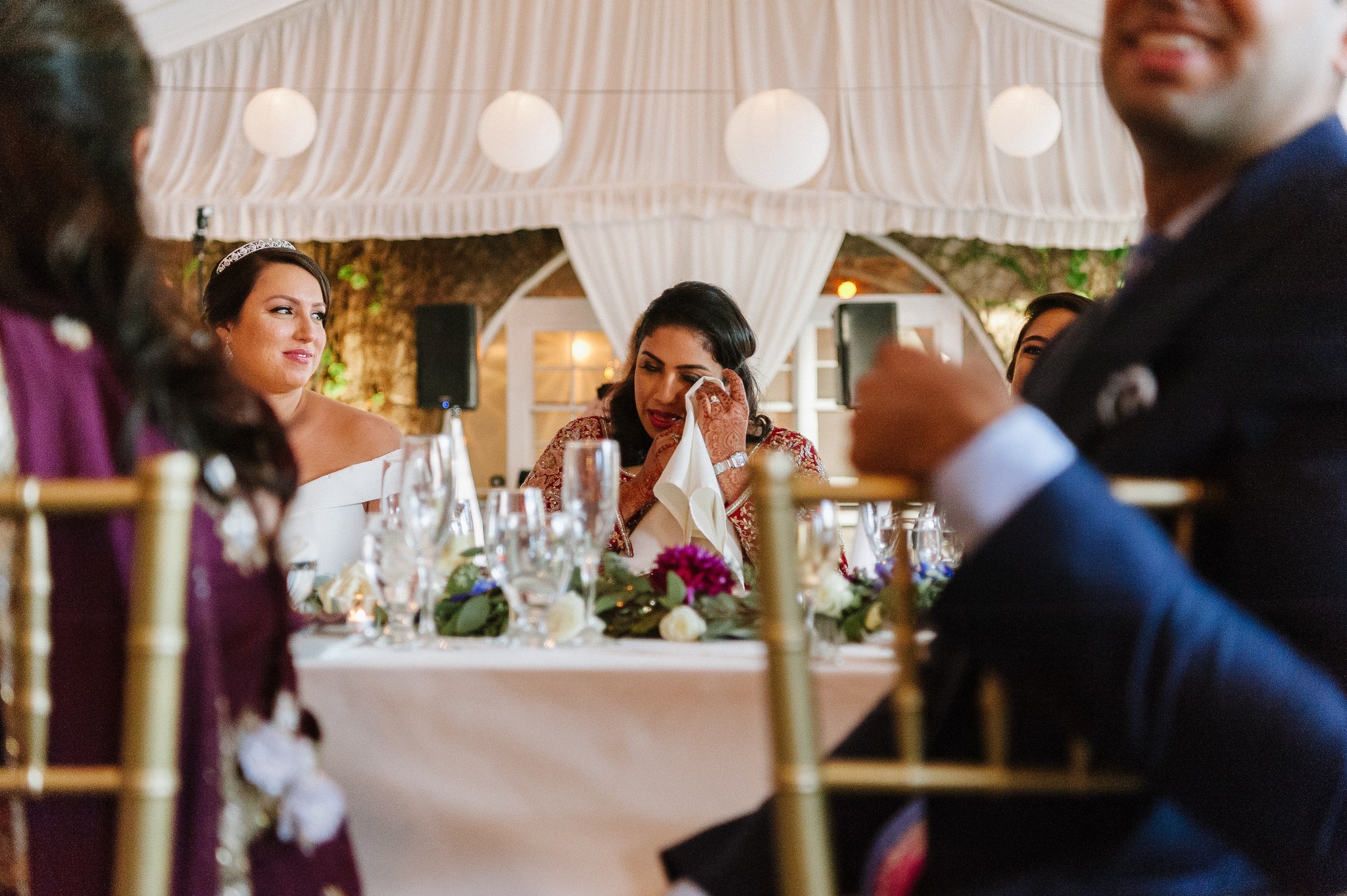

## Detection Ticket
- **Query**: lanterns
[477,90,562,173]
[243,87,318,160]
[988,87,1061,158]
[725,89,829,192]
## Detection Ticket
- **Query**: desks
[287,622,937,896]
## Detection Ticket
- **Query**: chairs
[0,448,200,896]
[748,449,1201,896]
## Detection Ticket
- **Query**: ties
[1114,226,1176,300]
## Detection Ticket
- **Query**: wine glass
[794,476,837,659]
[364,432,621,649]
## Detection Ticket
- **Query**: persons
[1007,292,1097,400]
[0,0,361,896]
[197,236,421,622]
[504,280,837,603]
[657,1,1347,896]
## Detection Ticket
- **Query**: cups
[859,500,963,589]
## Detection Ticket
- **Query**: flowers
[649,542,738,608]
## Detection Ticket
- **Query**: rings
[670,434,680,443]
[709,395,721,403]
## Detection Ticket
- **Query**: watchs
[713,451,749,476]
